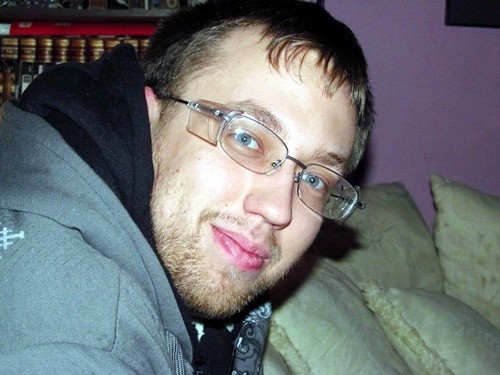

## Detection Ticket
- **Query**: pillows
[311,170,444,307]
[431,170,500,337]
[364,276,500,375]
[259,248,413,375]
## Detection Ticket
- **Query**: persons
[0,1,378,375]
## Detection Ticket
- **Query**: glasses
[160,96,367,222]
